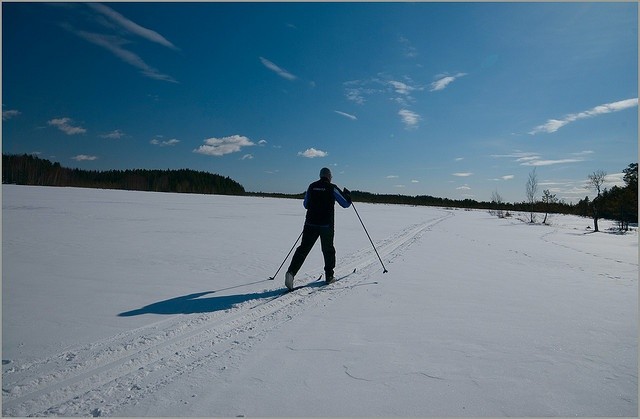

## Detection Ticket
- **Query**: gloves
[344,187,351,197]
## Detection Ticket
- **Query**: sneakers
[324,274,336,284]
[285,272,293,291]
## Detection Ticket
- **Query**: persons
[285,167,352,291]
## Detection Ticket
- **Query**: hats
[320,168,330,178]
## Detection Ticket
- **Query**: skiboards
[267,268,359,299]
